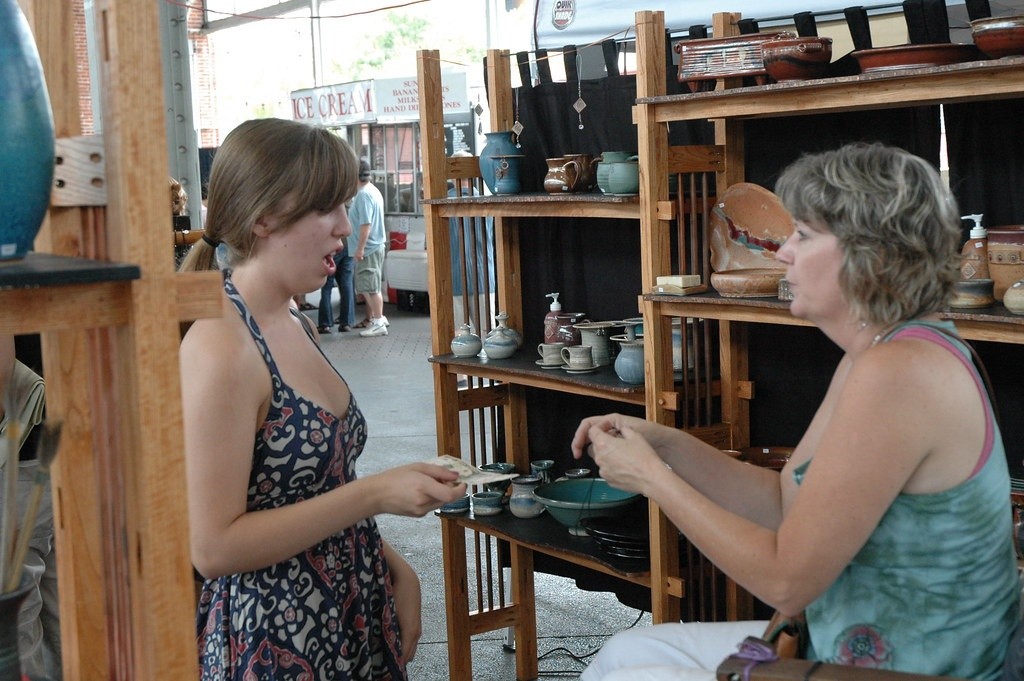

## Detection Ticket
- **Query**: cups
[535,318,704,384]
[544,150,639,194]
[440,460,591,518]
[986,225,1024,301]
[949,279,994,309]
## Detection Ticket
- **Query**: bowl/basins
[532,479,643,537]
[711,267,783,297]
[579,517,649,562]
[761,37,832,82]
[970,14,1024,59]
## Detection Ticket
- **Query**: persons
[168,178,366,333]
[342,159,390,338]
[573,144,1020,681]
[179,116,468,681]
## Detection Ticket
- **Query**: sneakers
[360,324,388,336]
[379,316,390,326]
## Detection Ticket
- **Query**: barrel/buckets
[390,231,406,249]
[407,232,425,251]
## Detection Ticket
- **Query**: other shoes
[334,316,340,323]
[357,318,369,328]
[338,324,351,332]
[316,325,331,333]
[298,302,314,310]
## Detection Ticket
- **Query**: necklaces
[867,328,889,347]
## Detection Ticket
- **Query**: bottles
[479,131,524,195]
[1003,276,1024,315]
[451,312,522,359]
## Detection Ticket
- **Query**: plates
[832,41,976,74]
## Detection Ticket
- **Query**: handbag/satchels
[761,604,806,660]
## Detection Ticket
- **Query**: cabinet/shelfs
[640,49,1024,347]
[415,9,742,680]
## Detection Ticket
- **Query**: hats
[357,160,370,176]
